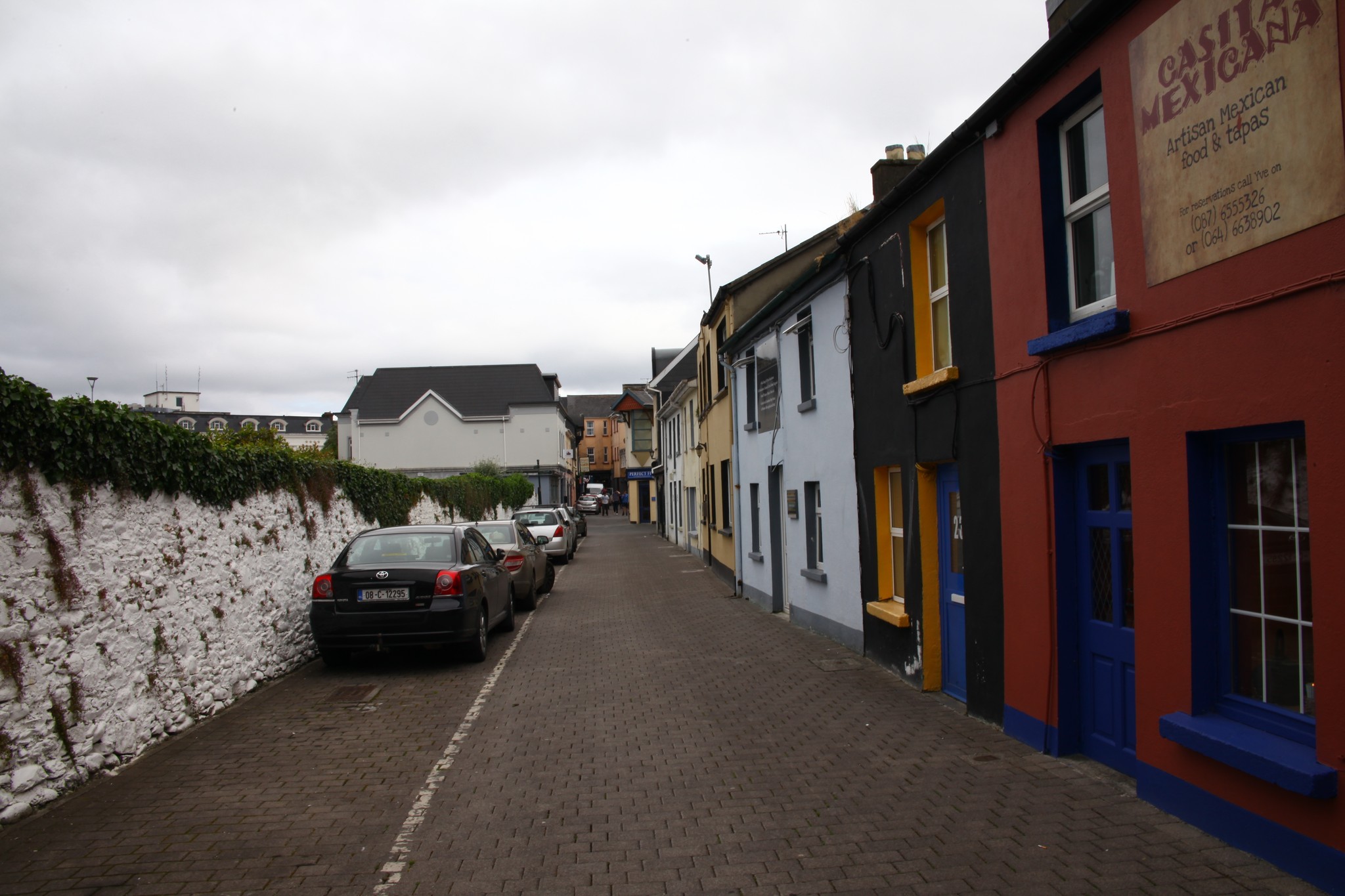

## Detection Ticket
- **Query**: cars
[575,493,602,515]
[567,506,588,539]
[435,519,555,611]
[528,503,579,552]
[509,504,573,565]
[308,523,517,663]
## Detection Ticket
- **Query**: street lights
[694,254,713,306]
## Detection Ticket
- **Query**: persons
[600,492,610,516]
[621,491,629,516]
[606,491,611,510]
[612,490,620,515]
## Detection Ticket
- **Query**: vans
[581,483,613,496]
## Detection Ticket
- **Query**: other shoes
[614,512,616,514]
[622,513,624,516]
[626,513,628,516]
[616,513,618,515]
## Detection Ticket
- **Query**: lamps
[695,442,707,458]
[649,449,656,459]
